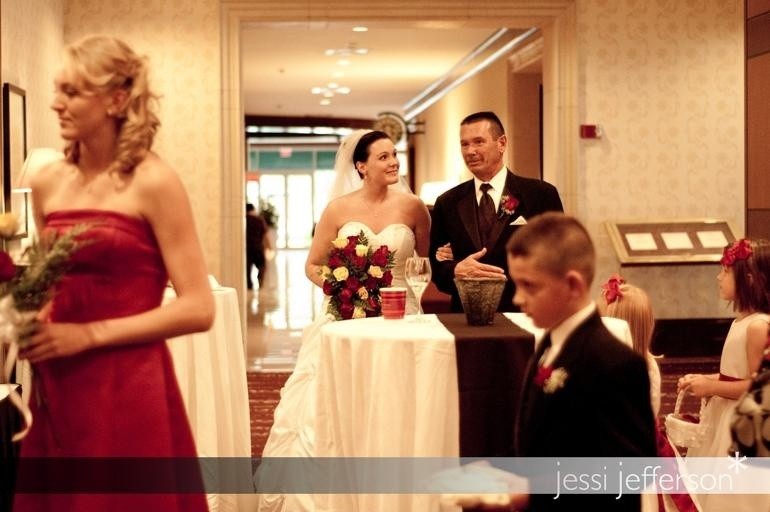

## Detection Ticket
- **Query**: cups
[378,287,408,320]
[451,277,508,326]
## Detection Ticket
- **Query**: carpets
[245,352,720,480]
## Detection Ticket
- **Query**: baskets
[665,384,707,449]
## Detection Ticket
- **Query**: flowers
[8,220,107,324]
[535,362,570,394]
[497,194,521,217]
[319,230,399,320]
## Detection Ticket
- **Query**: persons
[260,208,278,289]
[597,278,663,438]
[246,203,266,288]
[429,110,565,312]
[469,209,658,512]
[252,125,431,512]
[676,237,770,512]
[13,33,215,512]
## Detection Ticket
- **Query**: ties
[477,184,496,236]
[513,334,551,435]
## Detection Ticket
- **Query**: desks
[157,282,251,484]
[318,311,635,508]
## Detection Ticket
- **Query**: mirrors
[3,81,29,238]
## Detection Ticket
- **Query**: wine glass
[403,256,433,322]
[7,263,57,351]
